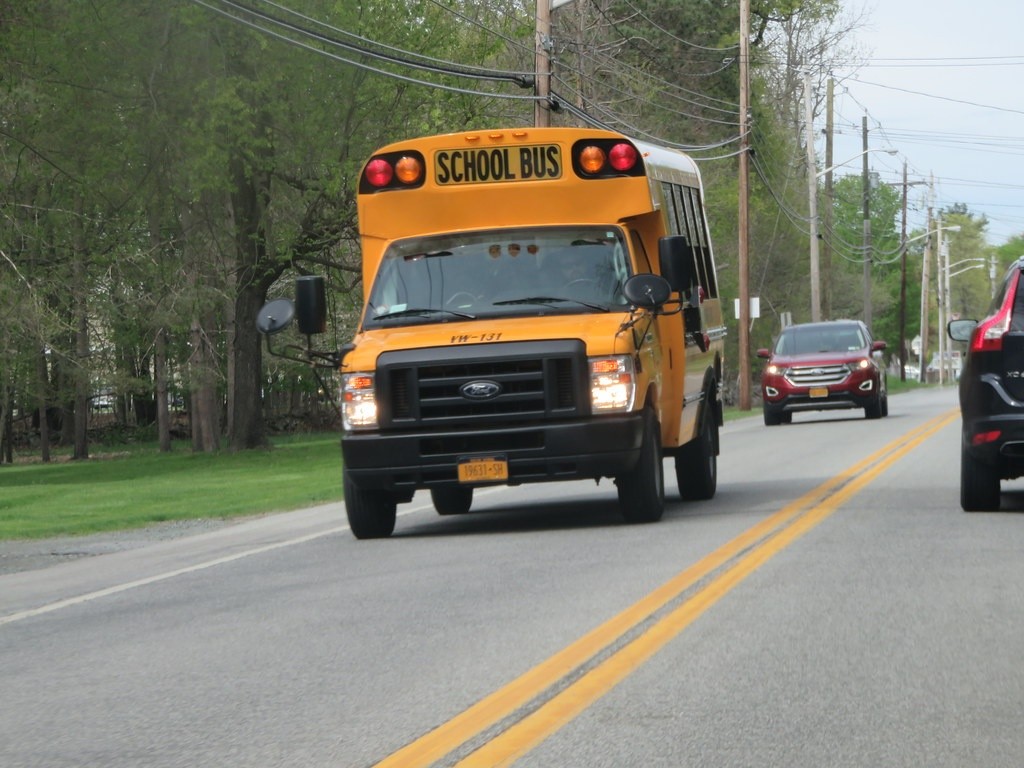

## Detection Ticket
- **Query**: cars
[904,350,962,381]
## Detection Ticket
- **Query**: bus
[255,128,723,540]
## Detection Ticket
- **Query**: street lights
[944,253,985,385]
[935,218,986,385]
[803,72,899,323]
[900,162,962,384]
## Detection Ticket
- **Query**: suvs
[947,256,1024,513]
[755,318,888,425]
